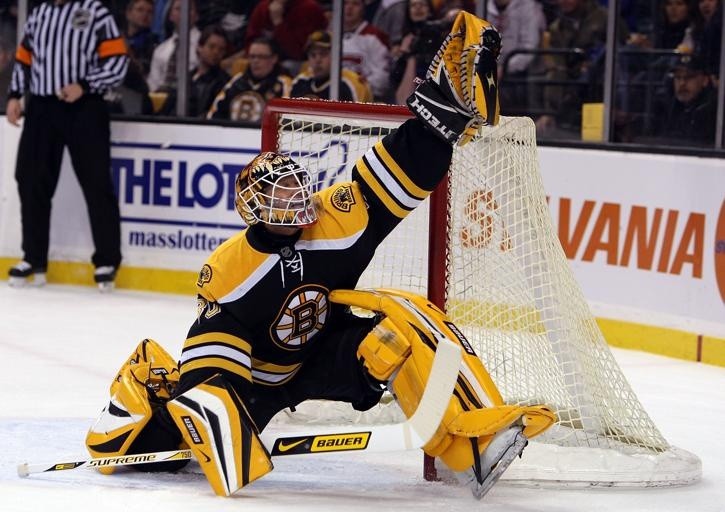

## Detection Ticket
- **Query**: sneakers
[94,266,116,282]
[455,427,522,486]
[10,261,48,276]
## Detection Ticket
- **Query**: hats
[669,55,699,75]
[304,30,333,51]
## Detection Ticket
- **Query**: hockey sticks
[17,338,462,478]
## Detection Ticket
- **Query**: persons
[85,13,554,487]
[110,1,406,123]
[387,1,725,146]
[3,0,131,284]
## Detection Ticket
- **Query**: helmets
[235,151,318,229]
